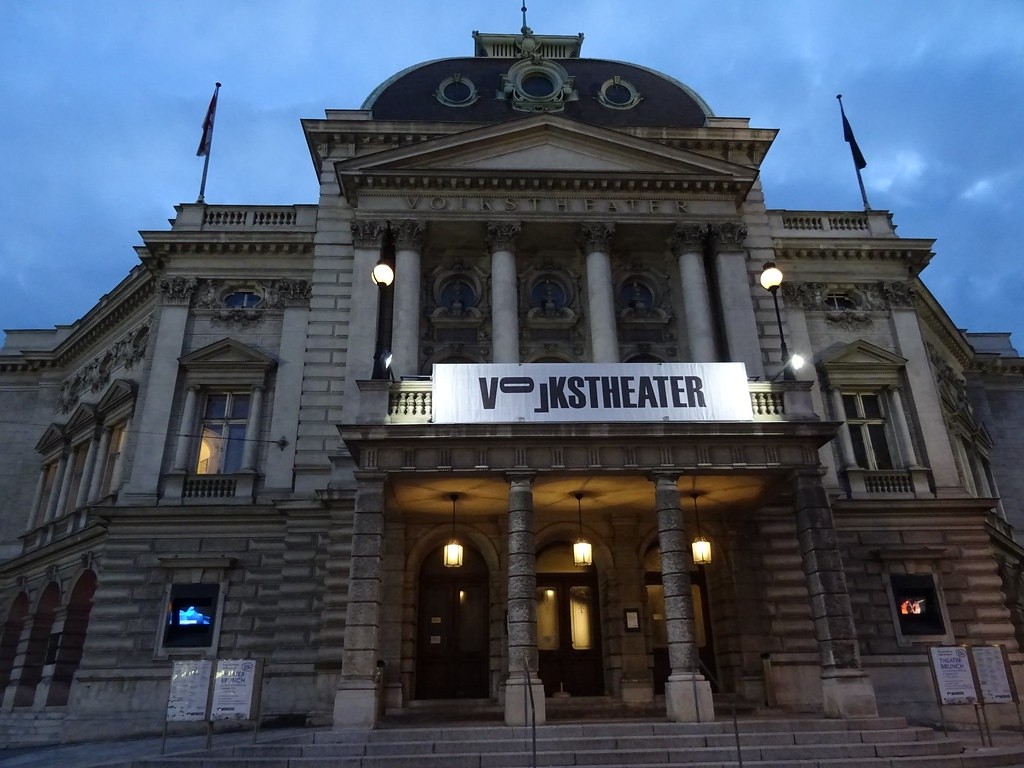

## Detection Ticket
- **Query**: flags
[840,110,866,170]
[195,87,219,156]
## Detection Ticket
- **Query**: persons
[541,278,561,318]
[448,280,468,319]
[625,281,651,319]
